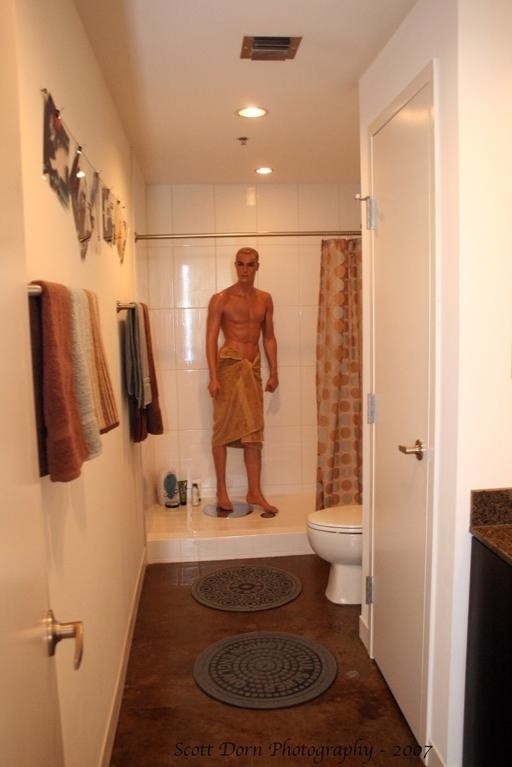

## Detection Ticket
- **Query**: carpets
[192,563,302,611]
[194,630,338,710]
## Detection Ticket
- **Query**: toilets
[306,503,363,605]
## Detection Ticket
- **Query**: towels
[29,281,119,482]
[211,346,265,449]
[124,302,164,443]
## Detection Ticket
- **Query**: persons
[206,247,279,514]
